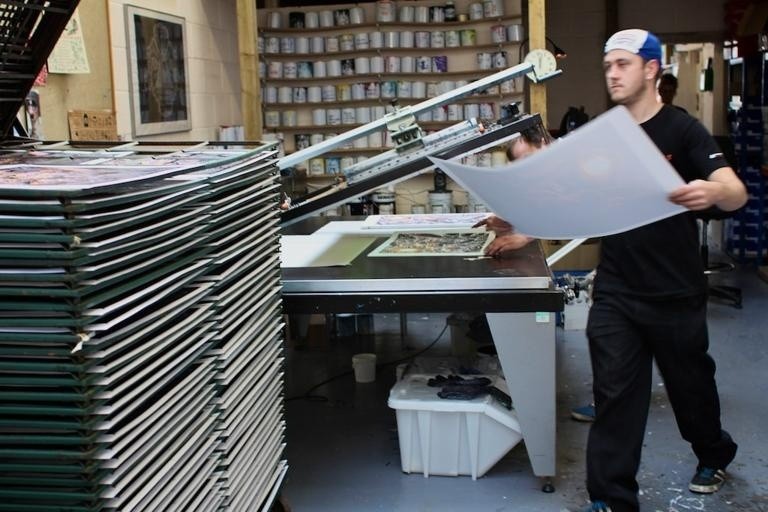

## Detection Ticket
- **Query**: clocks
[524,49,557,81]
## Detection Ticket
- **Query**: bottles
[433,167,447,192]
[445,2,455,22]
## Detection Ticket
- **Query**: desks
[281,213,566,493]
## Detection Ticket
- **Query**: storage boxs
[387,373,522,480]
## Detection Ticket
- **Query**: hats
[603,28,661,71]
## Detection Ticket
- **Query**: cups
[350,352,377,384]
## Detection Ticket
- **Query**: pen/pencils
[471,219,487,228]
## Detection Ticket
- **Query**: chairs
[695,135,743,310]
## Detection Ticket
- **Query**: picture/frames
[124,3,192,137]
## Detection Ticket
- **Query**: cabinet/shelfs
[235,0,546,213]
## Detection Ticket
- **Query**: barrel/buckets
[219,125,245,140]
[346,191,488,215]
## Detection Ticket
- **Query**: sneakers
[560,501,612,512]
[570,400,598,423]
[686,459,728,495]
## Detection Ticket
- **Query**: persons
[658,73,690,113]
[472,122,598,423]
[487,27,749,510]
